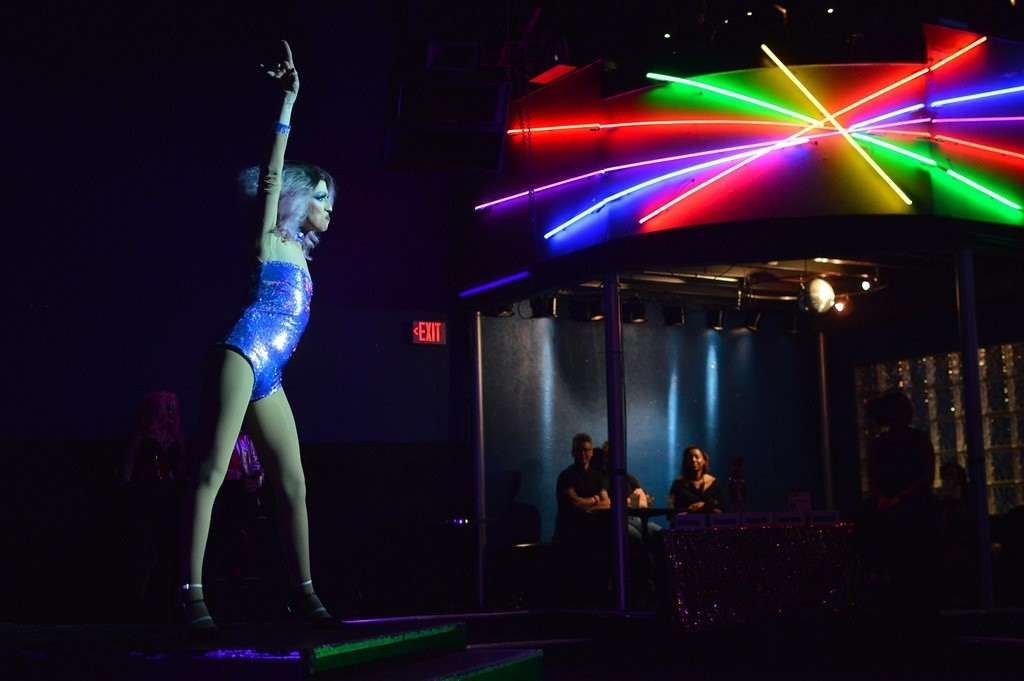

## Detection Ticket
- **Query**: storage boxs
[787,492,825,512]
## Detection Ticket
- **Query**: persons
[866,393,970,640]
[183,39,346,642]
[553,434,726,612]
[119,391,271,625]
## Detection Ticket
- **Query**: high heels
[288,588,344,627]
[181,600,223,642]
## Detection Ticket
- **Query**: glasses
[573,448,593,452]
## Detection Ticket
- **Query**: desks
[653,523,855,605]
[590,508,672,610]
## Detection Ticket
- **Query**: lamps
[671,304,687,327]
[711,305,726,331]
[735,277,850,335]
[496,304,515,317]
[529,295,551,318]
[628,290,648,324]
[586,288,604,321]
